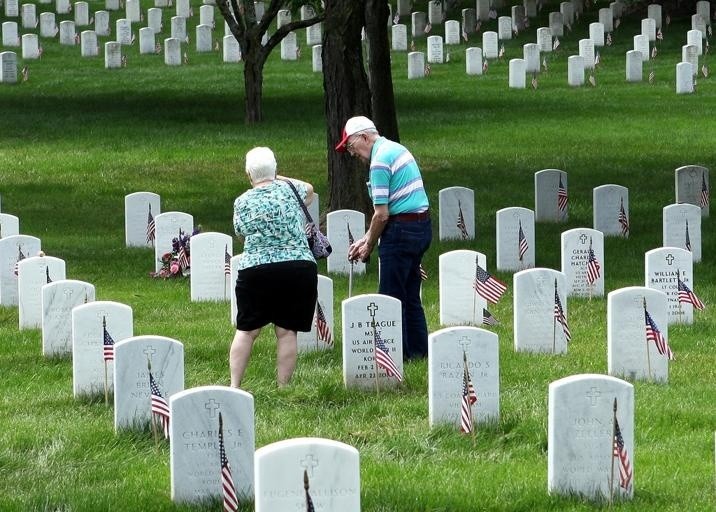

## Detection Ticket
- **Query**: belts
[391,214,428,221]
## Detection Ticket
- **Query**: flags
[618,203,630,237]
[456,208,469,242]
[701,174,710,210]
[348,229,360,265]
[471,265,508,303]
[145,212,155,244]
[558,178,570,214]
[177,238,190,271]
[685,226,692,252]
[586,246,603,287]
[613,413,634,490]
[460,365,478,435]
[16,3,194,84]
[646,308,675,363]
[373,326,403,384]
[517,227,531,261]
[418,262,428,283]
[13,250,26,276]
[149,371,169,441]
[103,327,115,360]
[209,3,318,59]
[218,424,240,512]
[678,278,709,311]
[482,307,499,325]
[553,291,572,342]
[314,301,334,353]
[392,1,715,92]
[225,251,231,276]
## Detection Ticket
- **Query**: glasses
[347,136,360,152]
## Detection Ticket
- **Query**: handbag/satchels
[308,231,333,260]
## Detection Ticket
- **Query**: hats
[335,116,376,153]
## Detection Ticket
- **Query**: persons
[226,145,319,392]
[333,114,435,364]
